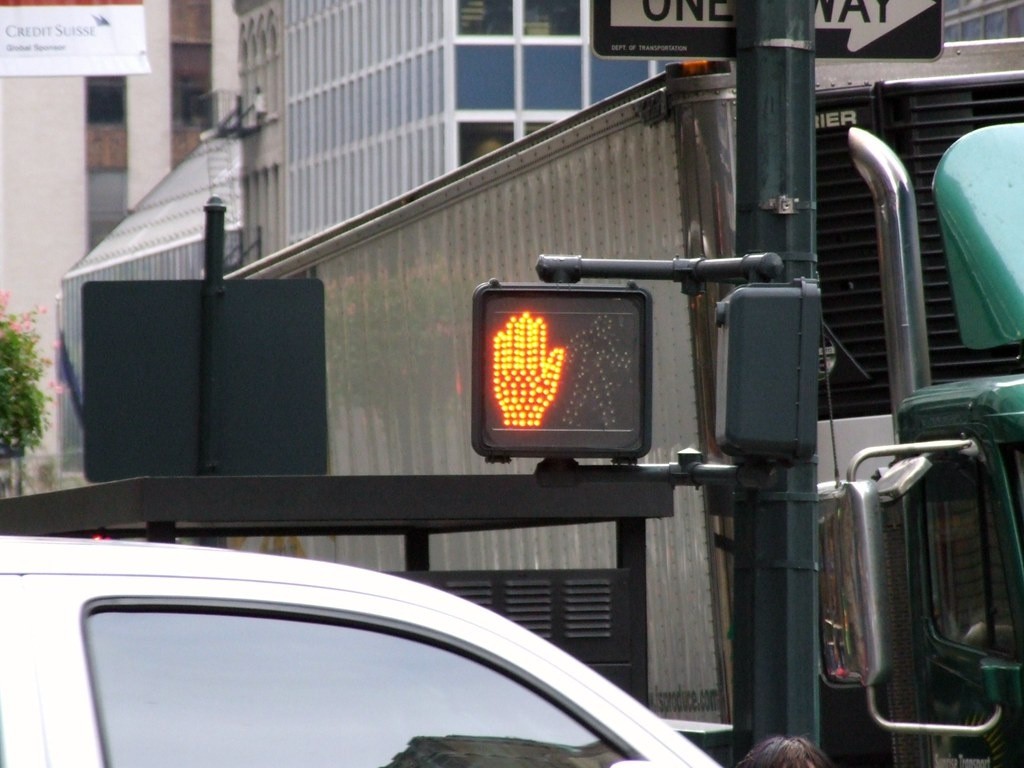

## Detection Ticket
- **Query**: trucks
[228,36,1022,766]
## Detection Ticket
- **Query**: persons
[735,735,835,768]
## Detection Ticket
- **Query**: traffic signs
[587,1,944,64]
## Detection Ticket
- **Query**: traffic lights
[467,283,653,459]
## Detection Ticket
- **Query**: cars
[1,529,724,766]
